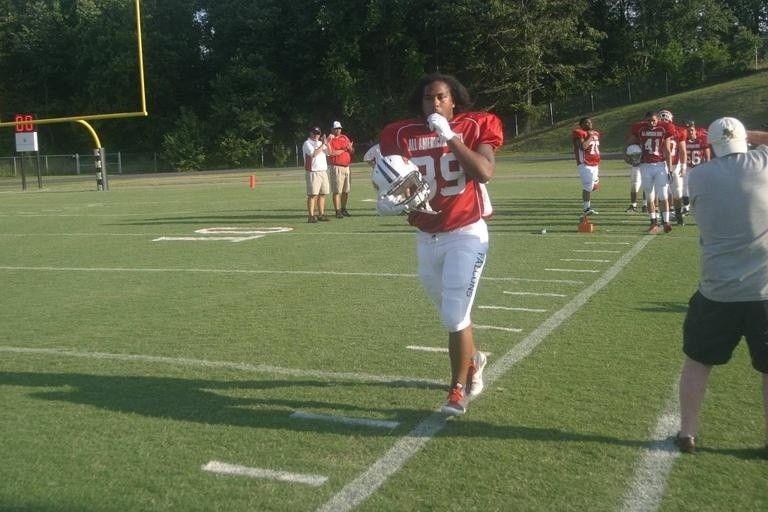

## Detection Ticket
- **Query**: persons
[674,116,768,454]
[326,121,355,218]
[302,126,332,223]
[378,72,504,414]
[623,109,711,234]
[570,117,601,215]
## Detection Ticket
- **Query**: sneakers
[466,350,488,397]
[673,429,696,453]
[583,205,691,236]
[305,208,352,225]
[438,379,469,418]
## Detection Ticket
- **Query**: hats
[705,116,751,160]
[329,120,343,130]
[310,126,321,136]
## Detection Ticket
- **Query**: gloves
[426,112,459,145]
[374,193,412,218]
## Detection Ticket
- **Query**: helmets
[657,109,674,123]
[625,145,644,167]
[369,155,431,213]
